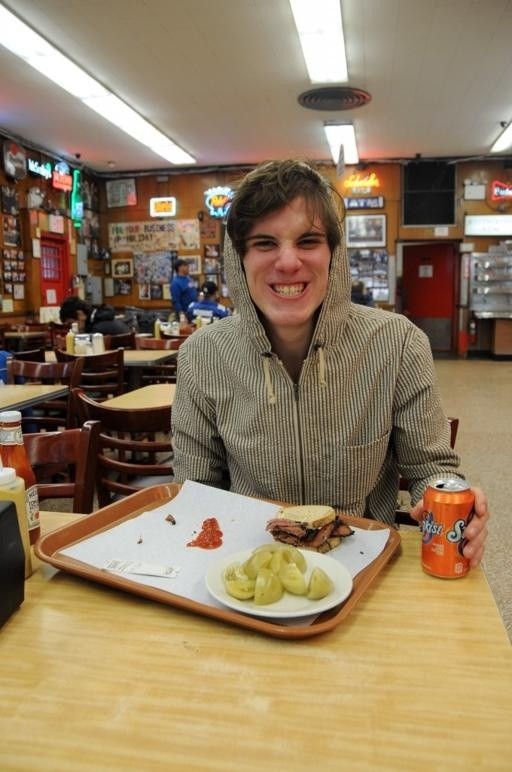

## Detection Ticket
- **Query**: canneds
[420,480,475,579]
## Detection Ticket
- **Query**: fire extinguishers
[458,329,466,357]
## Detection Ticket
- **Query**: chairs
[361,415,459,534]
[0,318,187,513]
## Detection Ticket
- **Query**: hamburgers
[265,504,354,553]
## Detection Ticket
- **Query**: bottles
[197,313,204,329]
[0,409,43,578]
[65,329,76,355]
[154,318,162,339]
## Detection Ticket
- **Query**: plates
[206,548,354,617]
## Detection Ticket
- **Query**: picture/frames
[137,283,153,300]
[176,255,203,275]
[109,259,134,279]
[343,212,389,248]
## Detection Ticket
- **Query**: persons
[169,258,199,325]
[166,157,492,573]
[186,281,233,326]
[59,295,133,351]
